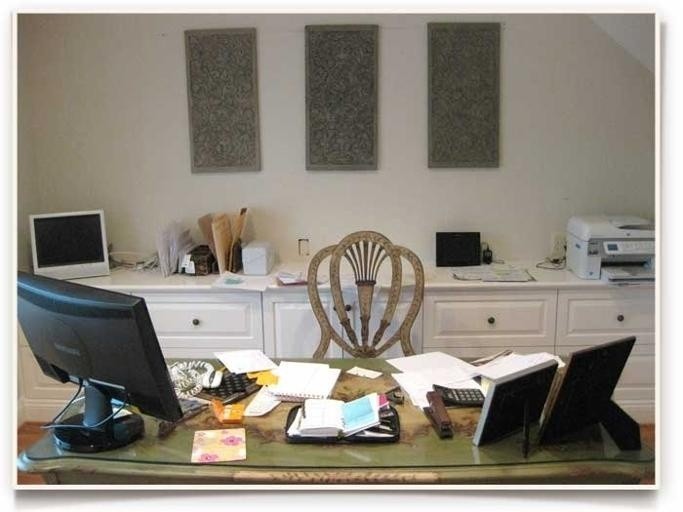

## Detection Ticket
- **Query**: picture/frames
[305,25,378,172]
[185,26,261,175]
[426,22,501,169]
[536,336,637,447]
[473,358,558,447]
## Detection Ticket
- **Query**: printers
[565,212,655,281]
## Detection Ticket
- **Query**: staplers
[425,394,453,436]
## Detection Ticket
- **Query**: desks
[19,357,654,486]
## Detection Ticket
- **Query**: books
[284,392,397,440]
[195,373,263,405]
[269,366,341,400]
[275,271,307,286]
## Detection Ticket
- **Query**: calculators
[434,385,484,408]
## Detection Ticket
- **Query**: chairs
[307,231,423,358]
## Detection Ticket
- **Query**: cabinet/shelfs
[557,288,655,425]
[263,295,424,360]
[129,292,263,361]
[422,289,557,361]
[17,318,87,423]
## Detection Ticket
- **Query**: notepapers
[221,405,244,423]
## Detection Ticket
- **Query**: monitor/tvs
[29,209,110,280]
[17,272,183,454]
